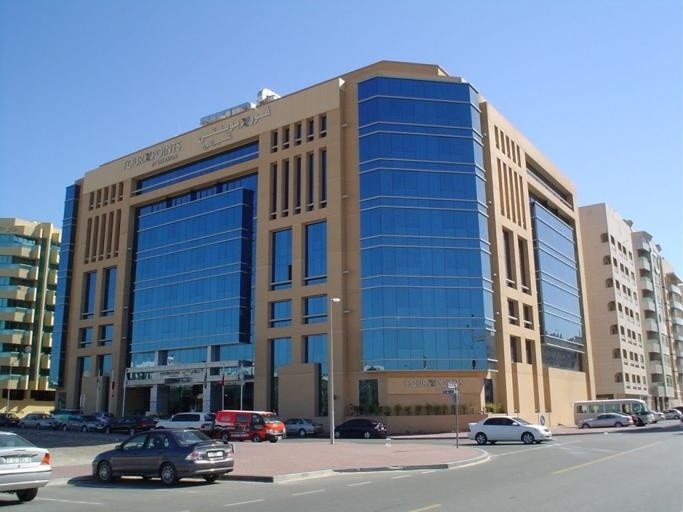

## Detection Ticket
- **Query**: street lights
[328,297,341,444]
[7,346,31,414]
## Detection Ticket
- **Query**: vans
[211,409,286,442]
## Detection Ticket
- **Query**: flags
[204,368,207,388]
[239,363,244,386]
[123,373,128,387]
[221,368,225,385]
[97,371,101,392]
[112,375,115,390]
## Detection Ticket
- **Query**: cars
[0,429,51,503]
[466,415,553,444]
[333,418,387,439]
[0,410,215,436]
[284,417,323,436]
[577,408,682,429]
[91,428,235,484]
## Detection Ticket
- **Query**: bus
[572,399,648,426]
